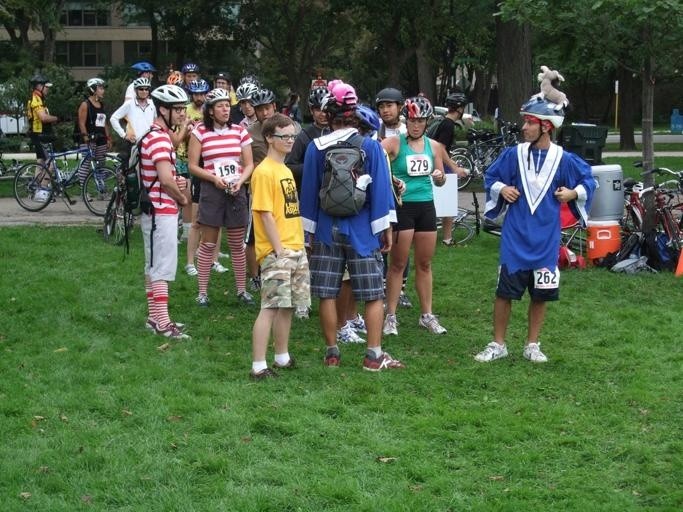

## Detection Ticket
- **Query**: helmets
[320,84,358,115]
[150,84,188,107]
[445,92,468,106]
[86,78,105,95]
[357,105,380,131]
[519,97,566,128]
[307,88,327,107]
[134,77,151,88]
[204,87,231,105]
[375,87,403,104]
[239,74,256,84]
[188,78,210,93]
[215,72,232,83]
[236,83,258,101]
[182,63,199,73]
[167,70,184,86]
[250,89,276,107]
[131,62,157,72]
[29,74,52,88]
[402,97,434,118]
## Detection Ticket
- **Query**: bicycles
[13,134,117,217]
[449,117,521,191]
[104,153,135,246]
[623,162,683,250]
[436,192,481,245]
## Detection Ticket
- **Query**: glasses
[271,133,296,141]
[173,106,187,113]
[138,88,149,91]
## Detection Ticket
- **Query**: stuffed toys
[531,66,568,112]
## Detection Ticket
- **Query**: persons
[327,80,344,92]
[335,105,405,345]
[214,71,230,90]
[429,93,468,248]
[283,92,301,124]
[249,113,310,387]
[238,73,258,86]
[245,88,300,291]
[76,78,112,202]
[182,64,201,93]
[230,87,243,123]
[125,63,156,101]
[379,98,448,336]
[185,78,229,258]
[27,74,57,203]
[331,84,358,105]
[171,120,199,243]
[284,88,331,203]
[167,68,183,86]
[186,103,229,276]
[298,96,405,371]
[374,88,413,313]
[237,83,259,128]
[472,94,595,364]
[109,78,156,143]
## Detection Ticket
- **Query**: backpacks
[116,130,172,267]
[72,98,94,143]
[317,135,366,217]
[427,118,449,138]
[286,110,297,120]
[190,156,202,204]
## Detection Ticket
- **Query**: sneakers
[154,322,192,341]
[97,191,111,201]
[211,262,229,273]
[180,235,188,242]
[362,348,404,371]
[250,276,260,292]
[383,313,399,336]
[337,323,366,345]
[198,293,208,308]
[237,291,255,306]
[474,341,508,364]
[323,346,341,368]
[249,368,278,381]
[295,306,310,319]
[347,313,367,334]
[218,252,229,259]
[146,317,185,332]
[441,238,463,249]
[184,264,198,276]
[35,189,56,204]
[523,341,547,363]
[272,358,296,370]
[80,192,93,203]
[397,295,412,307]
[177,236,182,245]
[419,312,447,335]
